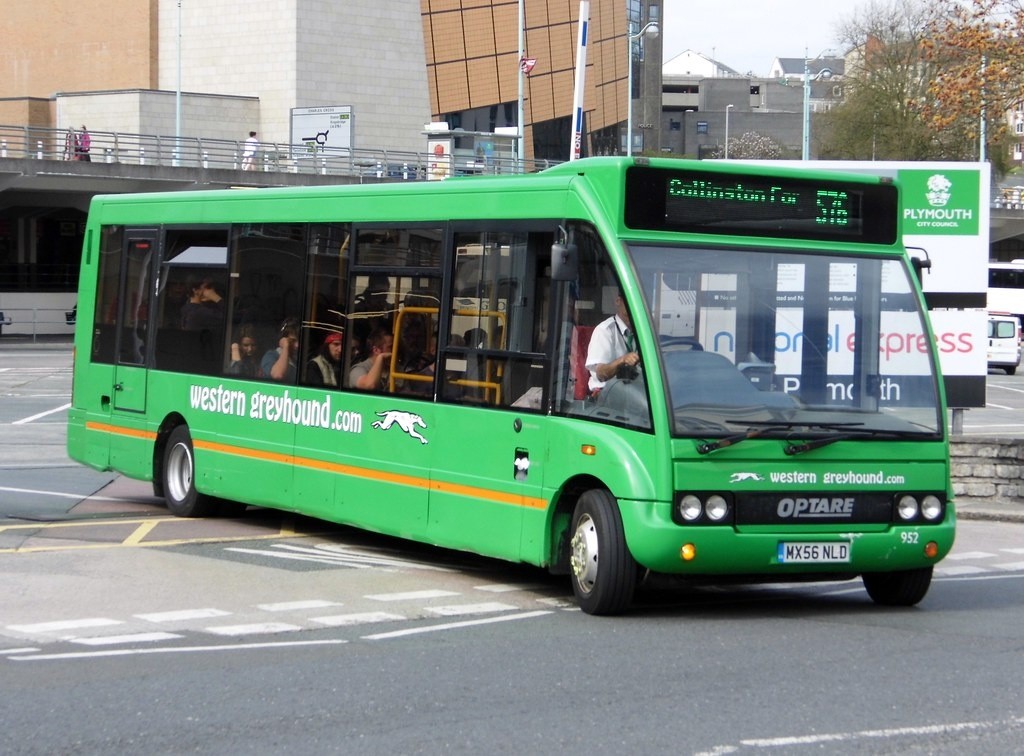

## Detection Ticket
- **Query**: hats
[325,331,342,344]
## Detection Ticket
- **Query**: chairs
[567,324,598,404]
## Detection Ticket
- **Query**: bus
[67,155,956,620]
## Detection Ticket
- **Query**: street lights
[724,104,738,162]
[627,21,660,159]
[801,60,833,160]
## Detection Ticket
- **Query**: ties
[625,331,634,353]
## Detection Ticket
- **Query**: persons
[415,328,502,402]
[178,273,224,329]
[242,132,260,170]
[350,331,411,393]
[353,276,437,328]
[259,319,300,384]
[303,332,342,388]
[65,126,91,161]
[230,322,258,375]
[583,294,641,392]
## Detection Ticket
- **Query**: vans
[987,315,1022,375]
